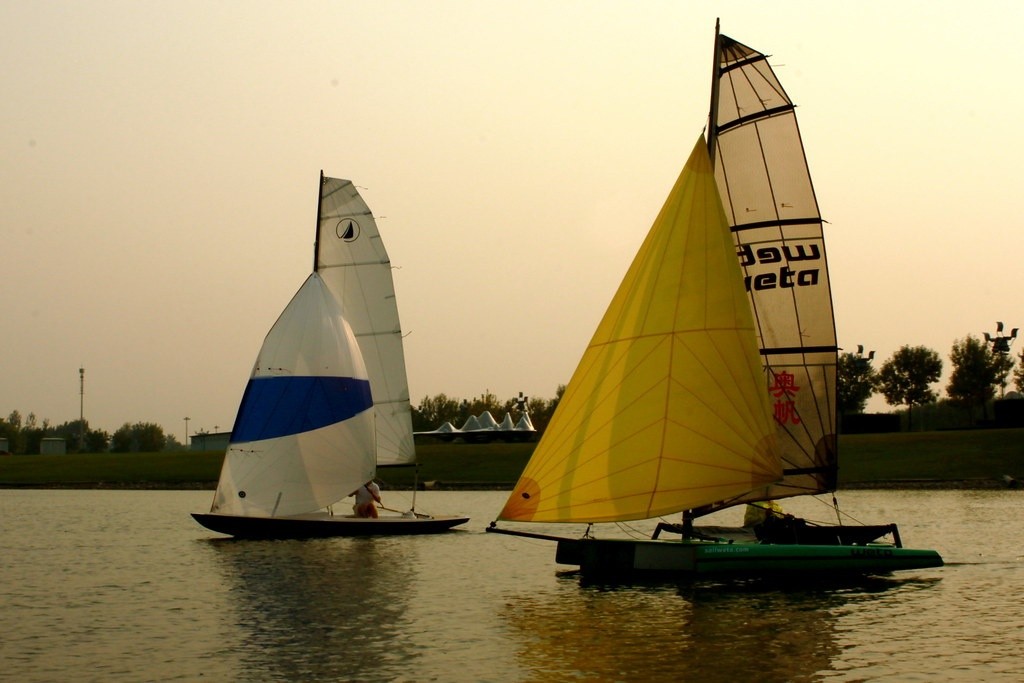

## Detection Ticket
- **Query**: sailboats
[189,168,470,536]
[485,16,946,590]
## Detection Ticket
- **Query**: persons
[348,480,381,519]
[744,501,794,527]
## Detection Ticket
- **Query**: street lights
[183,417,191,446]
[983,322,1019,400]
[78,365,84,452]
